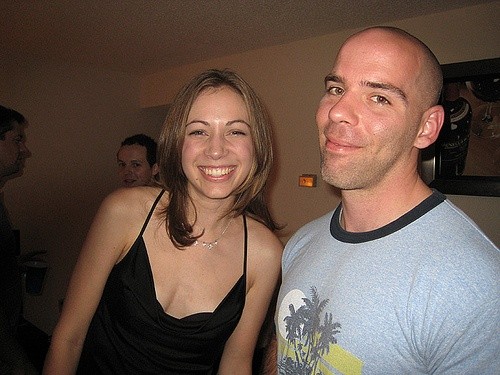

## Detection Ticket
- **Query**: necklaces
[184,210,234,251]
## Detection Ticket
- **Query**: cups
[23,262,48,295]
[465,77,500,138]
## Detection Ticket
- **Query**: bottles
[436,82,472,178]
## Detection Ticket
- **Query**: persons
[0,105,34,375]
[41,68,284,375]
[275,25,500,375]
[116,134,161,187]
